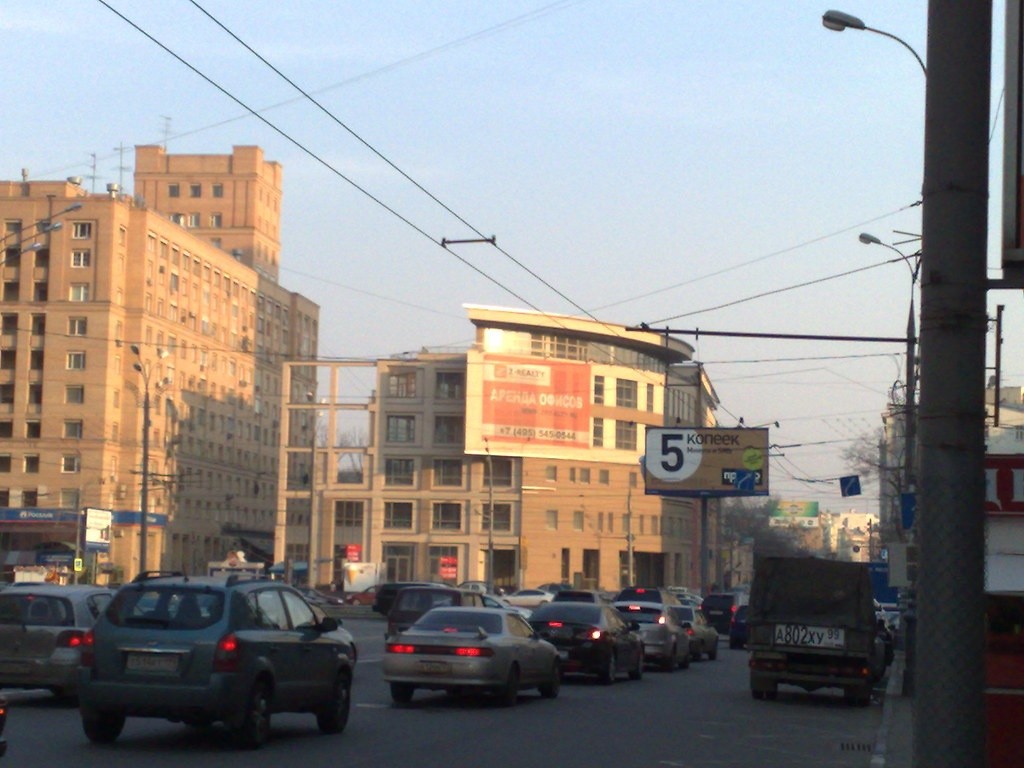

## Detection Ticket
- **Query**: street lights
[857,231,916,699]
[130,345,168,572]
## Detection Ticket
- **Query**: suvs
[76,570,359,750]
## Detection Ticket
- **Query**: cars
[372,580,720,672]
[0,581,154,708]
[382,605,564,707]
[527,600,645,685]
[730,603,896,667]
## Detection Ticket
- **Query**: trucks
[749,553,884,708]
